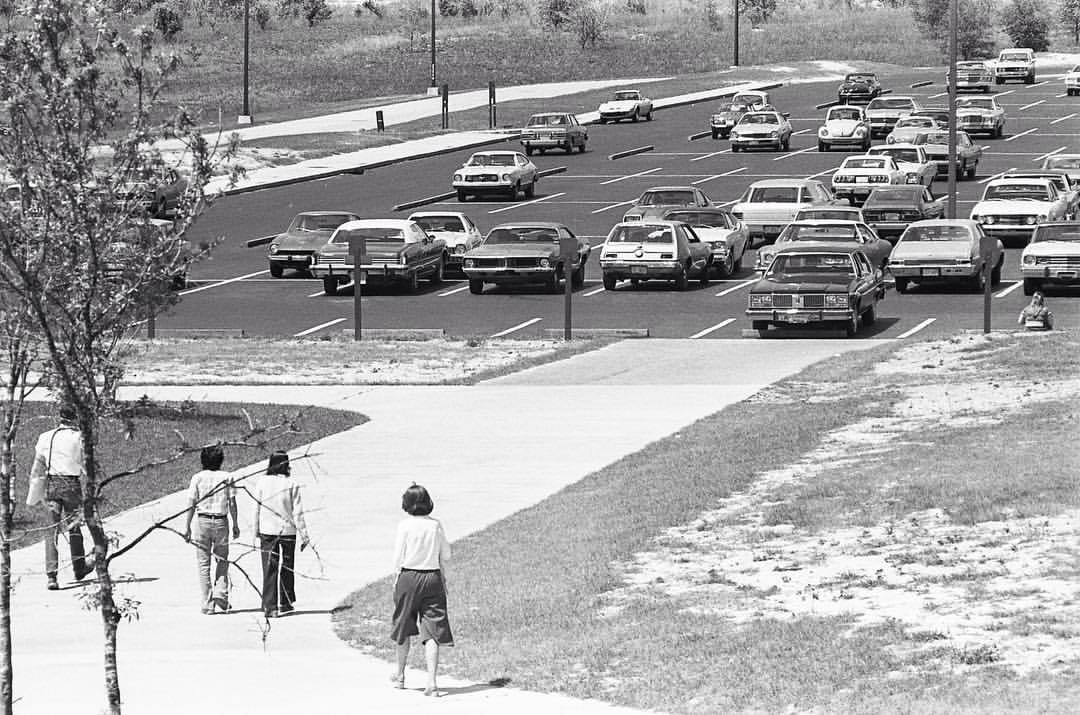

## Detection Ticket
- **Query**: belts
[198,513,227,519]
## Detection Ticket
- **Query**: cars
[266,210,360,277]
[945,61,994,93]
[967,170,1080,242]
[518,111,589,156]
[708,90,986,286]
[308,218,450,296]
[952,95,1006,139]
[658,206,751,276]
[84,167,194,219]
[1064,63,1080,97]
[406,211,484,278]
[598,220,715,291]
[461,220,592,295]
[1019,220,1080,298]
[618,185,715,223]
[451,150,541,201]
[885,218,1005,292]
[88,216,193,291]
[598,88,655,124]
[745,243,882,337]
[836,72,881,105]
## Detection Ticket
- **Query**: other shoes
[280,603,295,612]
[76,559,95,580]
[47,577,59,590]
[203,609,214,614]
[213,595,232,610]
[264,608,277,617]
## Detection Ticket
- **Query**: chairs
[444,222,460,230]
[537,234,553,241]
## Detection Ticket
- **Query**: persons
[184,445,241,615]
[387,485,456,698]
[252,450,309,617]
[29,404,95,589]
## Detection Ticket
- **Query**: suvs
[994,48,1038,85]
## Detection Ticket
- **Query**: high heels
[389,672,405,689]
[424,686,438,697]
